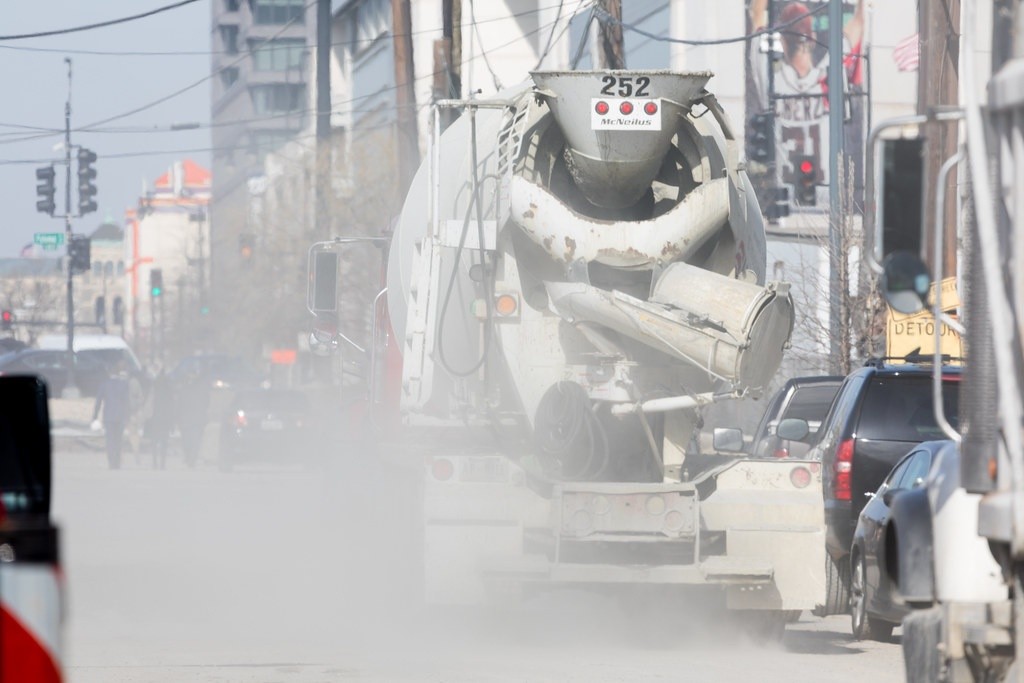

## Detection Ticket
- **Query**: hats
[773,4,813,36]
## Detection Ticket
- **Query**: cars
[222,388,314,445]
[0,349,106,394]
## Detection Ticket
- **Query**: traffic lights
[36,166,55,217]
[2,311,12,332]
[199,294,210,317]
[78,149,97,214]
[150,271,162,296]
[794,153,816,207]
[765,183,790,219]
[750,111,772,164]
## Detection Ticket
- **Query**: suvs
[775,352,964,615]
[713,374,846,459]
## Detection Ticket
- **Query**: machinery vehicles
[901,55,1024,683]
[307,65,797,636]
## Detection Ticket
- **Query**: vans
[38,334,145,383]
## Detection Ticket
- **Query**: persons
[89,364,206,472]
